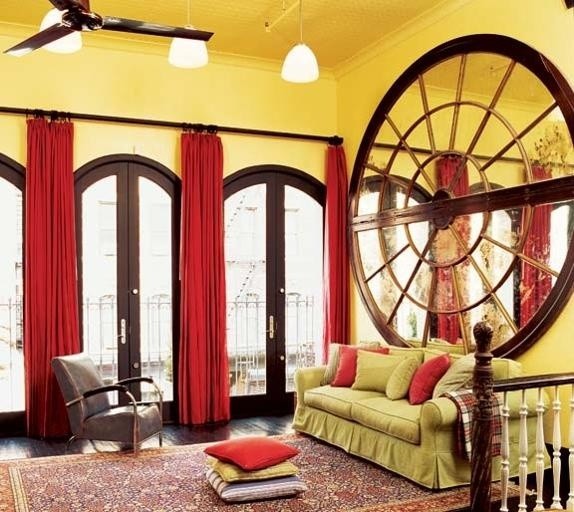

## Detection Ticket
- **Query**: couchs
[287,357,551,493]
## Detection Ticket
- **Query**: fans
[0,0,213,58]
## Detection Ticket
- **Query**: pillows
[201,436,307,504]
[320,342,477,406]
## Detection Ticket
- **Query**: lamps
[280,3,319,85]
[167,0,208,67]
[37,8,83,54]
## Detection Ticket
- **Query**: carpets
[0,433,515,510]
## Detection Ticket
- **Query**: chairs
[50,352,164,454]
